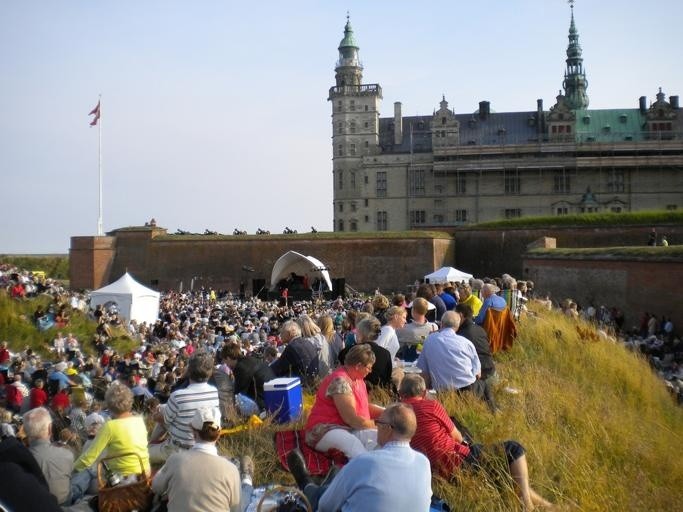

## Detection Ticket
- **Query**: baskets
[97,452,154,512]
[256,486,313,512]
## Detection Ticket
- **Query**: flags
[87,99,100,126]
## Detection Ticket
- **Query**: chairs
[402,304,437,324]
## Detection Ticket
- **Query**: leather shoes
[287,449,311,488]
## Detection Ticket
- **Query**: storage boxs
[261,377,301,423]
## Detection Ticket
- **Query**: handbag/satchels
[235,392,260,417]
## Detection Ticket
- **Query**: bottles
[101,460,121,486]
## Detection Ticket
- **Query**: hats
[191,404,222,430]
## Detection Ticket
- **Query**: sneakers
[241,455,255,481]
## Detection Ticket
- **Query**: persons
[0,220,682,512]
